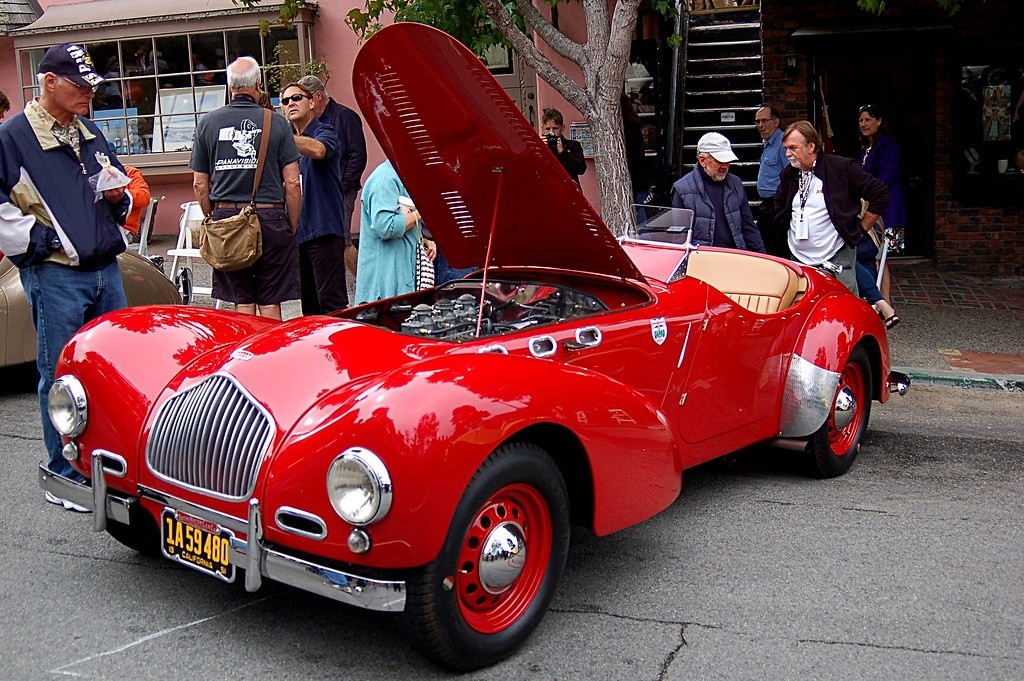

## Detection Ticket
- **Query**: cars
[0,236,194,382]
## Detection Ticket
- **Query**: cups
[998,160,1008,173]
[399,195,414,216]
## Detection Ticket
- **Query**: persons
[297,75,368,290]
[0,44,134,512]
[188,56,302,322]
[280,83,349,316]
[755,104,791,259]
[192,54,214,86]
[352,158,480,307]
[107,141,150,244]
[774,120,889,298]
[855,261,900,331]
[620,92,653,225]
[0,91,10,125]
[857,104,905,320]
[670,132,766,254]
[542,108,587,192]
[103,49,173,109]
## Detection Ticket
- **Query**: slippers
[885,312,900,329]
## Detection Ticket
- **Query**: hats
[697,131,739,163]
[37,42,105,87]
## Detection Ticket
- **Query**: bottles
[115,137,134,155]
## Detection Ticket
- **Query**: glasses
[754,116,774,124]
[59,76,100,93]
[859,104,877,110]
[282,93,310,105]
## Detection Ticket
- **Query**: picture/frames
[151,84,227,153]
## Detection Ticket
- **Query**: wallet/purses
[415,243,436,290]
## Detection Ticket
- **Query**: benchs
[686,250,799,314]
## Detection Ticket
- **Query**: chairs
[168,199,225,310]
[129,196,159,257]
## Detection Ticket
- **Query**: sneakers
[43,491,92,515]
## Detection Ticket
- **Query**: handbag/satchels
[197,206,263,270]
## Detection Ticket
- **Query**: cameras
[542,132,560,147]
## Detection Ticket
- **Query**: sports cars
[38,21,911,675]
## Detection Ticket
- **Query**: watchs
[51,240,61,252]
